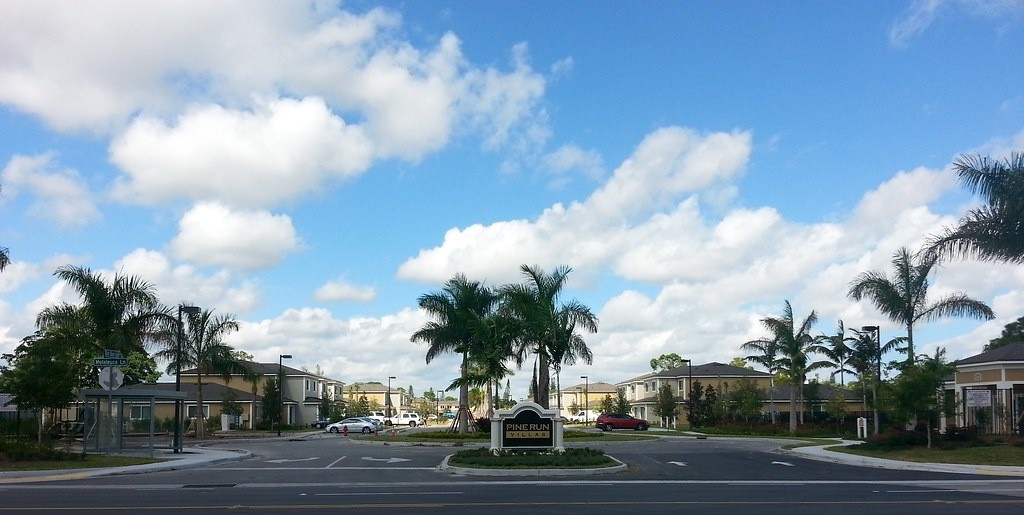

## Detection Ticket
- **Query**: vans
[384,412,420,428]
[572,409,601,425]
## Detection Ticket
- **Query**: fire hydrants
[343,425,349,436]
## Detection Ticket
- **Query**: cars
[363,410,393,426]
[597,413,650,432]
[326,416,381,434]
[426,413,438,422]
[311,417,333,430]
[443,409,457,419]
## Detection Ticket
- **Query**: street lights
[861,325,881,382]
[174,304,202,453]
[388,376,396,418]
[581,376,588,426]
[680,359,693,430]
[278,354,292,436]
[436,390,443,424]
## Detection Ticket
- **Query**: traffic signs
[92,357,129,367]
